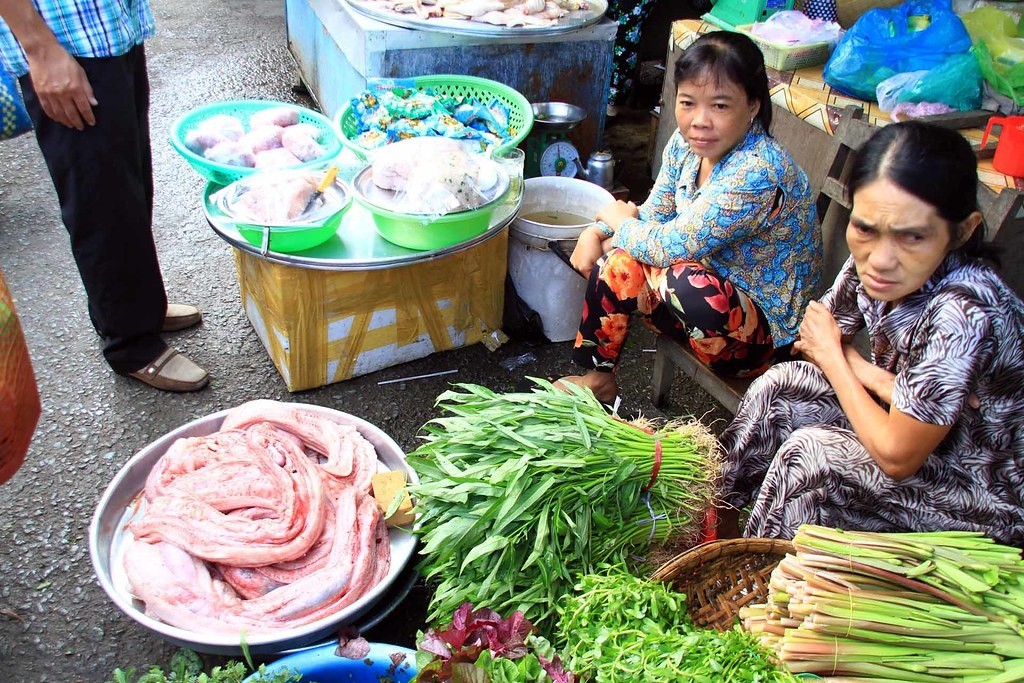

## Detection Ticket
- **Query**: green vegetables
[108,644,307,683]
[400,376,1024,683]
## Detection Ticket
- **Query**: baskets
[337,72,534,165]
[169,99,344,187]
[641,536,796,633]
[734,20,831,71]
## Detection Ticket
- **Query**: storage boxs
[231,227,511,391]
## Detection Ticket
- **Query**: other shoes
[128,346,207,391]
[530,372,609,403]
[161,303,203,330]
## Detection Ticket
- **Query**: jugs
[572,148,616,192]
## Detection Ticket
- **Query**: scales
[523,101,589,178]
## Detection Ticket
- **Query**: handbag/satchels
[823,0,1024,113]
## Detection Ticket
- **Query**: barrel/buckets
[507,177,616,343]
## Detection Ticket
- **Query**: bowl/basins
[89,403,422,655]
[231,176,352,252]
[350,184,508,251]
[242,643,422,683]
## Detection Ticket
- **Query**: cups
[979,115,1024,179]
[492,147,526,201]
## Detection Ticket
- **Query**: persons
[516,30,824,405]
[710,119,1023,549]
[0,0,209,393]
[604,0,655,131]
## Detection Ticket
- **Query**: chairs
[653,102,1023,422]
